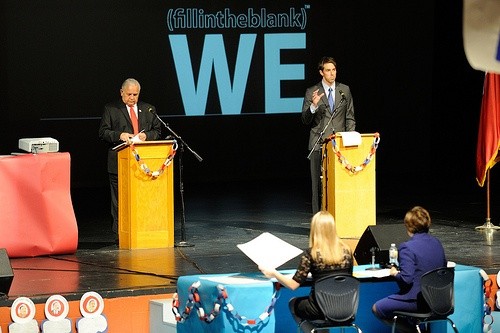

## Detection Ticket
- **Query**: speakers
[353,224,411,265]
[0,248,15,298]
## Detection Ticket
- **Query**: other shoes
[110,243,118,250]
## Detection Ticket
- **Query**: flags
[476,71,500,188]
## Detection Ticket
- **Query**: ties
[328,87,334,112]
[130,105,138,135]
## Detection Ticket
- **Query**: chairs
[391,267,459,333]
[300,273,362,333]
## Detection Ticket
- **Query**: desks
[177,264,483,333]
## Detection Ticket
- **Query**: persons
[258,211,351,333]
[100,78,161,241]
[301,56,355,220]
[372,206,444,333]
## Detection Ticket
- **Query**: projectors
[19,137,59,153]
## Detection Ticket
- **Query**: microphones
[148,107,156,115]
[339,90,347,101]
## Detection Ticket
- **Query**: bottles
[389,243,398,265]
